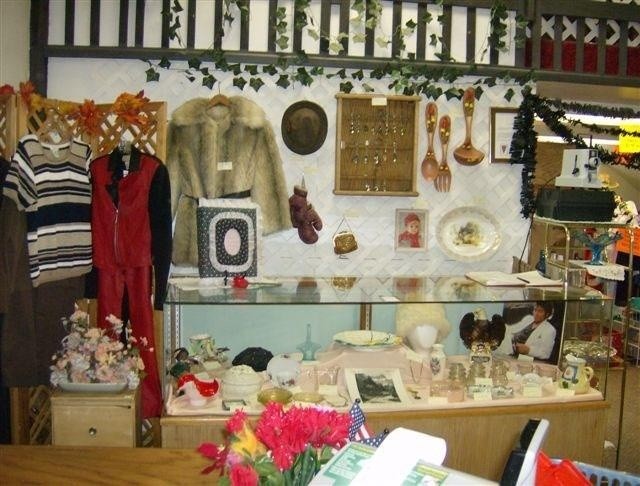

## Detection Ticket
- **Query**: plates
[434,205,504,264]
[434,275,497,314]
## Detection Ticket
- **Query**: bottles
[535,249,545,274]
[186,334,213,352]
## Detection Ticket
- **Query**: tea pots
[558,353,594,395]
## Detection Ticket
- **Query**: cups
[551,252,563,264]
[606,248,618,265]
[548,265,586,288]
[296,365,340,391]
[516,363,557,382]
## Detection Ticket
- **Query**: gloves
[297,203,323,244]
[289,185,308,228]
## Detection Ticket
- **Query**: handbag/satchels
[332,217,359,255]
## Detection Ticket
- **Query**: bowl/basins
[564,338,617,360]
[218,365,262,402]
[256,387,324,408]
[332,330,395,352]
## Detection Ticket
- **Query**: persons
[396,290,451,358]
[497,301,557,362]
[399,214,421,248]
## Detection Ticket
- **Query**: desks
[0,444,230,486]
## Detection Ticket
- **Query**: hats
[280,101,328,155]
[404,213,420,227]
[395,290,452,343]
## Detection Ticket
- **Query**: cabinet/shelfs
[157,270,618,486]
[47,385,145,449]
[531,216,635,472]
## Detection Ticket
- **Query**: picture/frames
[394,208,430,254]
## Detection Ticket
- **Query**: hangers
[202,77,233,112]
[118,130,135,164]
[31,111,74,148]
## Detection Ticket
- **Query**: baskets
[546,457,638,485]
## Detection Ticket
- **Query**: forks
[433,114,452,193]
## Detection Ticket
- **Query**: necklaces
[410,358,424,384]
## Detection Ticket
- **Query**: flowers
[191,398,356,486]
[47,307,155,393]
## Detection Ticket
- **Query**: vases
[55,380,128,393]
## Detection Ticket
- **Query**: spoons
[347,98,413,191]
[420,101,440,183]
